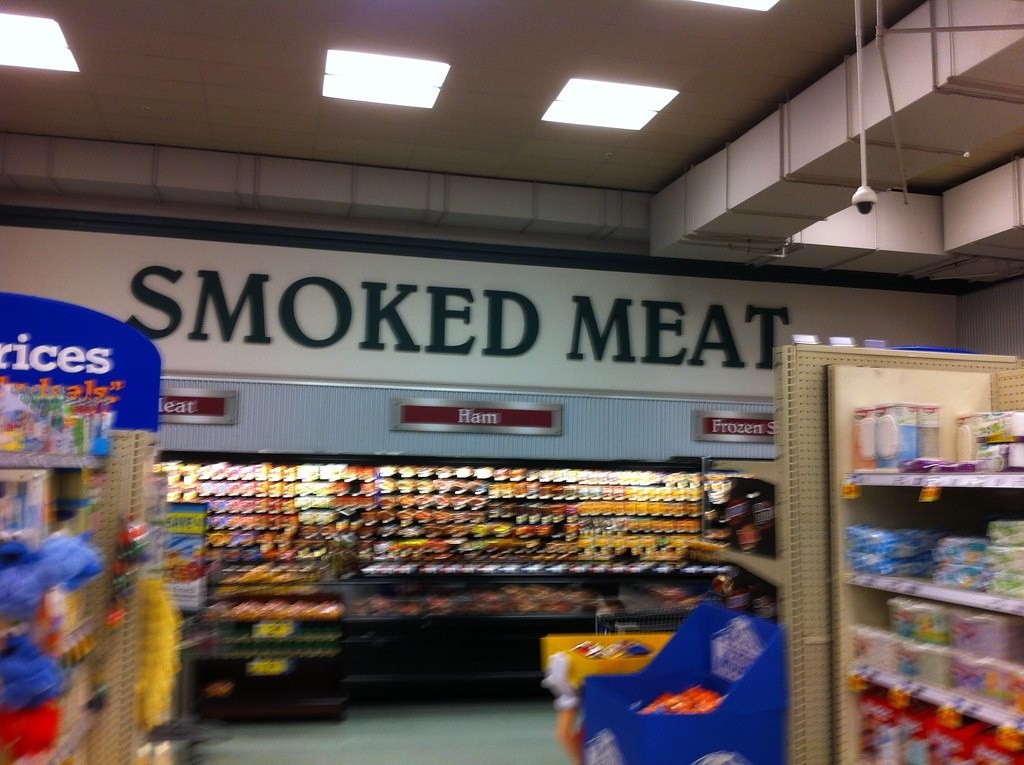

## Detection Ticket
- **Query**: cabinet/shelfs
[0,343,1024,765]
